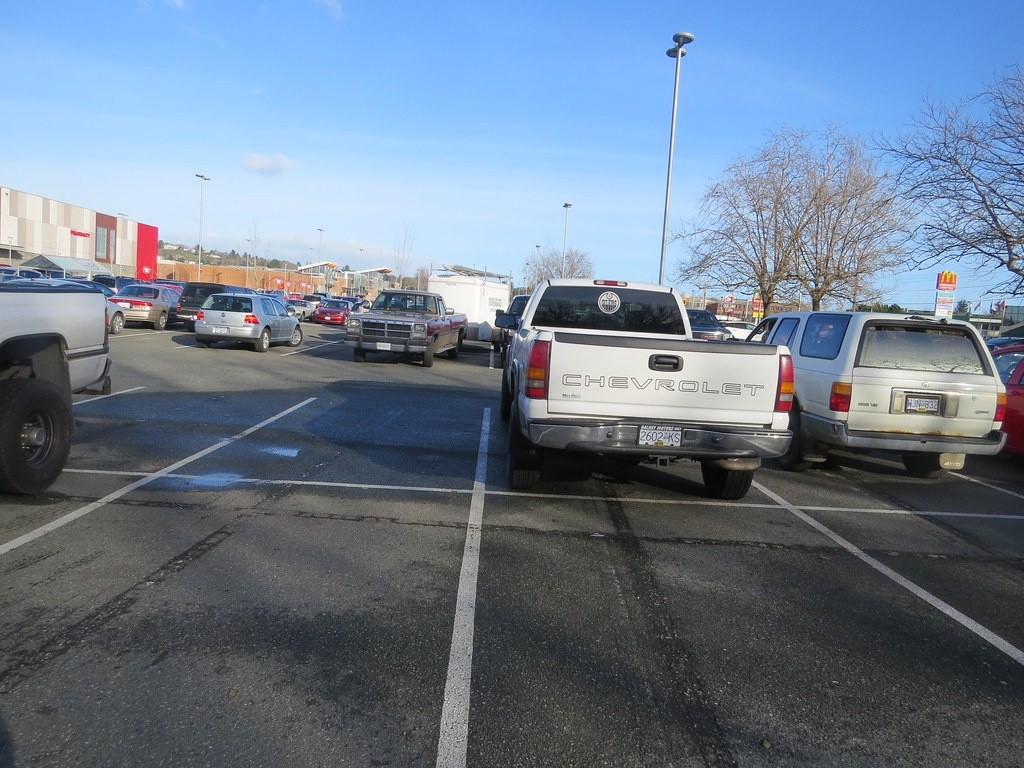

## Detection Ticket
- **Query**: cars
[255,288,396,328]
[0,262,188,337]
[495,295,533,368]
[673,308,768,342]
[194,292,303,353]
[980,335,1024,456]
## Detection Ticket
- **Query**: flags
[974,303,980,311]
[996,301,1004,312]
[990,303,994,313]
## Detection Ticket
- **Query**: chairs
[232,302,242,310]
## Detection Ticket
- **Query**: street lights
[310,248,313,285]
[246,239,251,288]
[195,174,210,282]
[657,32,694,288]
[359,249,364,287]
[560,202,572,278]
[535,245,541,287]
[316,228,325,291]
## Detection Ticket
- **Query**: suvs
[742,308,1007,478]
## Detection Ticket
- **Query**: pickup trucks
[494,278,795,500]
[343,289,469,368]
[0,282,112,495]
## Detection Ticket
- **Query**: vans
[176,281,260,329]
